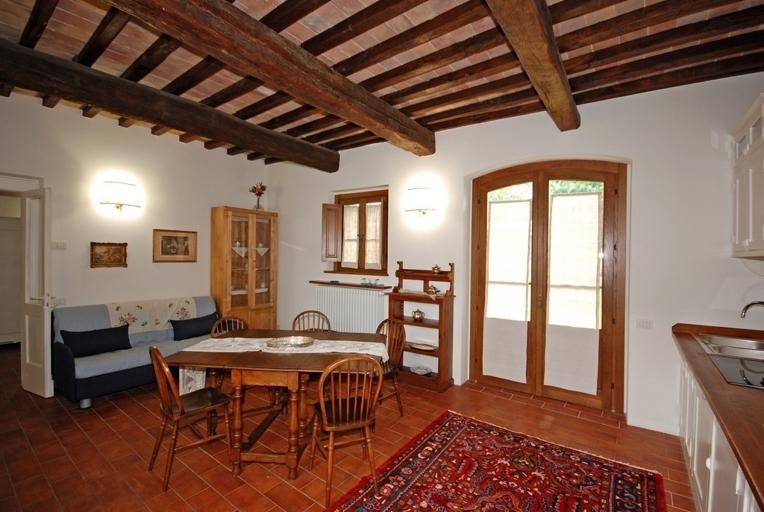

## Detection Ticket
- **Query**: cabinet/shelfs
[211,205,278,329]
[680,361,761,512]
[730,93,764,258]
[384,261,456,392]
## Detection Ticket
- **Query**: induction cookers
[706,352,763,388]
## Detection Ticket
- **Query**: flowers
[249,181,266,197]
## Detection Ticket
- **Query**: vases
[255,194,262,210]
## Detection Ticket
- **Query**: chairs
[148,346,232,492]
[211,316,248,395]
[372,319,406,418]
[292,310,330,332]
[309,355,384,508]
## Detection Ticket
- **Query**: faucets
[741,301,764,318]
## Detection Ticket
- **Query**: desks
[164,327,387,479]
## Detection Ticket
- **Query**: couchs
[50,295,222,409]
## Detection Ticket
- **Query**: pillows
[168,311,219,341]
[60,323,133,358]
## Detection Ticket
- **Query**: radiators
[312,285,384,334]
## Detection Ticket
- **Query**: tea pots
[412,309,425,321]
[424,285,441,295]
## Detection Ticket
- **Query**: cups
[234,241,240,248]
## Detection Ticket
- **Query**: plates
[266,335,315,348]
[409,366,433,376]
[409,342,437,352]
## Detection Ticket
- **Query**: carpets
[323,410,667,512]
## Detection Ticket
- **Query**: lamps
[404,187,436,216]
[99,181,141,210]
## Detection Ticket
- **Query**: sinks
[691,331,764,350]
[702,342,764,360]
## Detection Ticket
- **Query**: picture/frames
[153,228,198,263]
[90,241,128,268]
